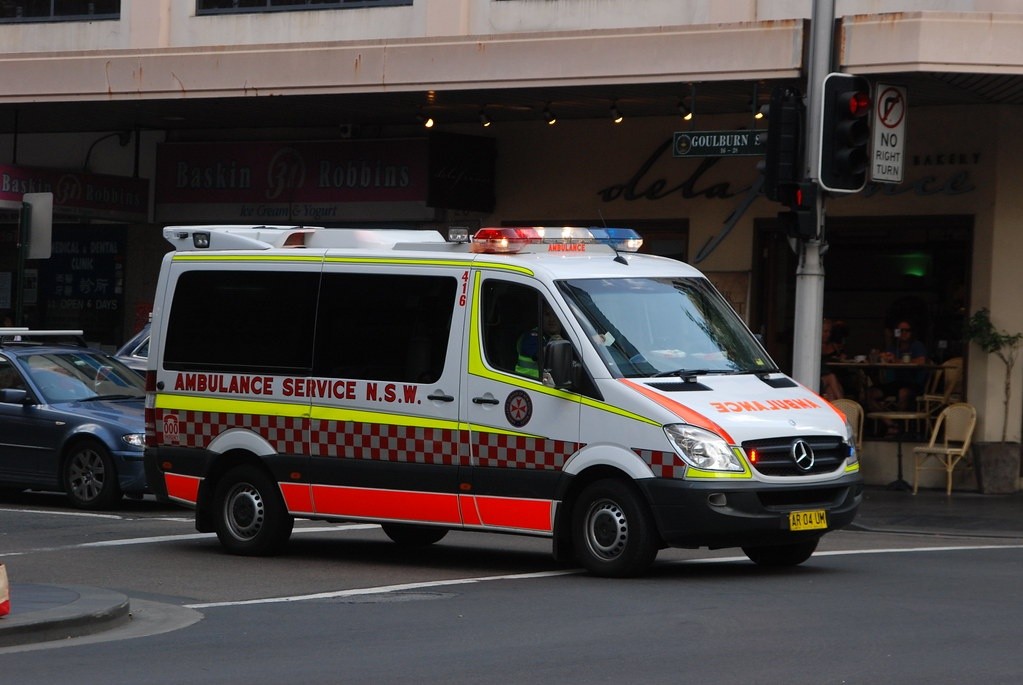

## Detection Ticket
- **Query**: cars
[70,313,153,387]
[1,326,145,513]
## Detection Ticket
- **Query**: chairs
[490,319,529,373]
[828,399,873,501]
[912,402,982,498]
[913,355,968,441]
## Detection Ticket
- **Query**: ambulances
[142,224,866,581]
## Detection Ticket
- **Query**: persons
[881,321,930,434]
[820,318,842,400]
[515,307,572,391]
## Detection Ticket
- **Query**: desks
[866,412,930,492]
[825,362,957,443]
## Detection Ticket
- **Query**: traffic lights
[776,179,821,242]
[816,69,873,194]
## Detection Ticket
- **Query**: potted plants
[961,308,1023,494]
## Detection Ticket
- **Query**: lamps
[747,100,763,121]
[542,100,556,127]
[479,103,492,128]
[608,96,623,124]
[416,112,436,128]
[677,93,693,121]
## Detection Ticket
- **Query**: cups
[856,355,866,362]
[901,353,912,363]
